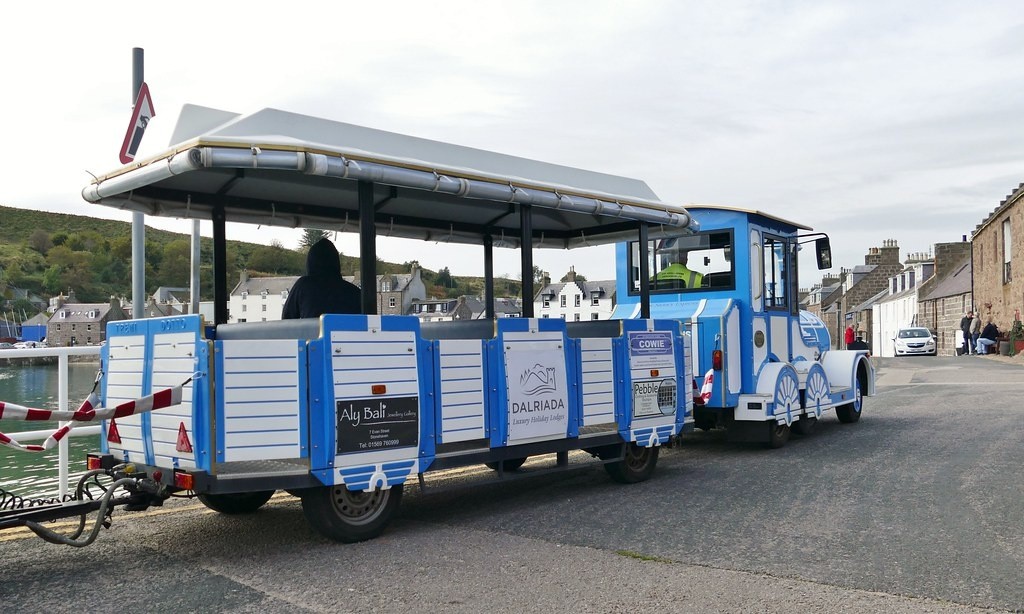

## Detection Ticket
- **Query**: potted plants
[1008,319,1024,356]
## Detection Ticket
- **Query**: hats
[973,312,980,316]
[666,253,688,263]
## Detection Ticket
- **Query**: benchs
[962,331,1012,355]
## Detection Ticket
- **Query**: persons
[649,252,707,289]
[845,323,855,350]
[960,311,998,356]
[281,238,361,320]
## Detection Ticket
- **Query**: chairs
[702,272,731,287]
[649,279,686,290]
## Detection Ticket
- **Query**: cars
[0,341,46,349]
[891,327,939,357]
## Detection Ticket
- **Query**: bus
[85,99,873,546]
